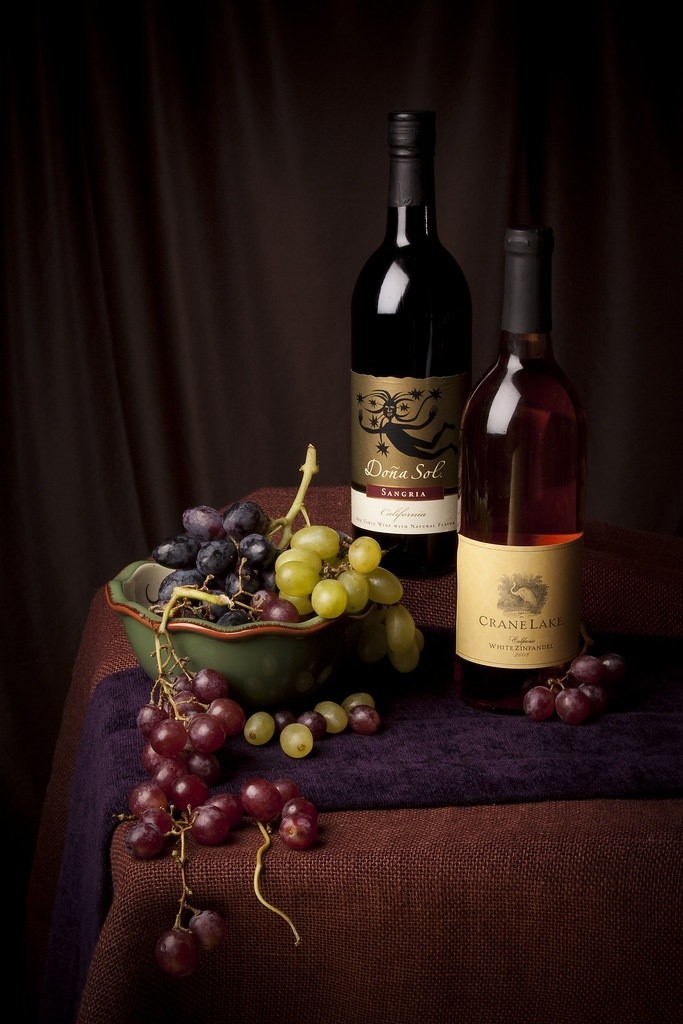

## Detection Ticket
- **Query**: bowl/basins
[105,561,374,710]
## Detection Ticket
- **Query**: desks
[25,484,683,1024]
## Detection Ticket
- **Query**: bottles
[451,225,585,715]
[350,111,471,579]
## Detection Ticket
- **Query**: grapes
[520,653,628,725]
[127,665,379,979]
[151,500,425,676]
